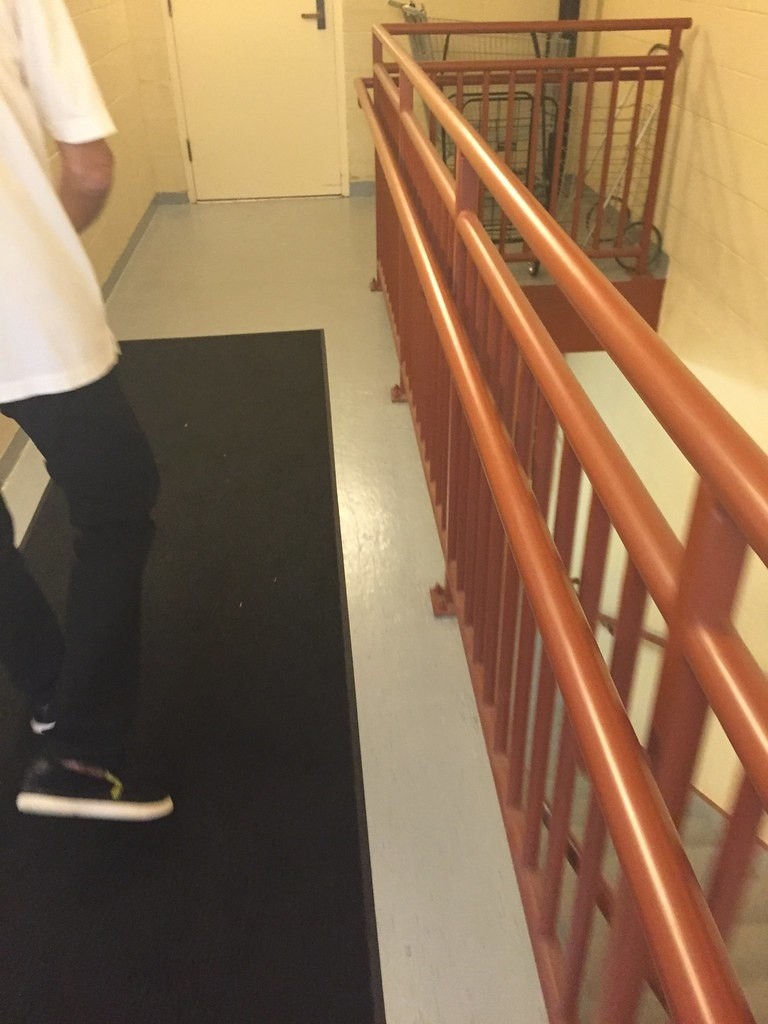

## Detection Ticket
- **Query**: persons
[0,0,176,820]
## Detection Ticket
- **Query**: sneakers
[17,746,172,822]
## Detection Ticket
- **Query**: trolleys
[387,1,684,272]
